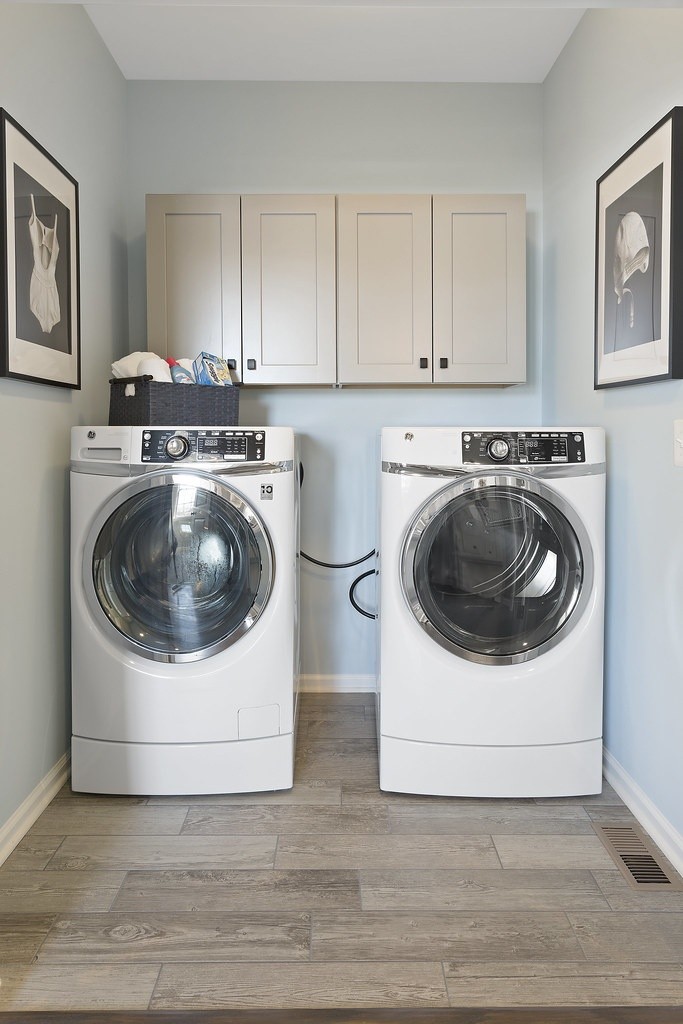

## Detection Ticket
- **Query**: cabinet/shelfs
[146,193,335,388]
[336,193,527,387]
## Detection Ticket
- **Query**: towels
[111,351,196,383]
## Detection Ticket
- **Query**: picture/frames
[594,106,683,390]
[0,107,81,389]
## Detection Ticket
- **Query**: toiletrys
[166,359,196,384]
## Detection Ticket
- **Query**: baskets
[108,375,245,426]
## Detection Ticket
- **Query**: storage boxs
[192,352,232,386]
[108,376,244,426]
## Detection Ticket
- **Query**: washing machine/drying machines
[72,429,303,796]
[378,421,609,794]
